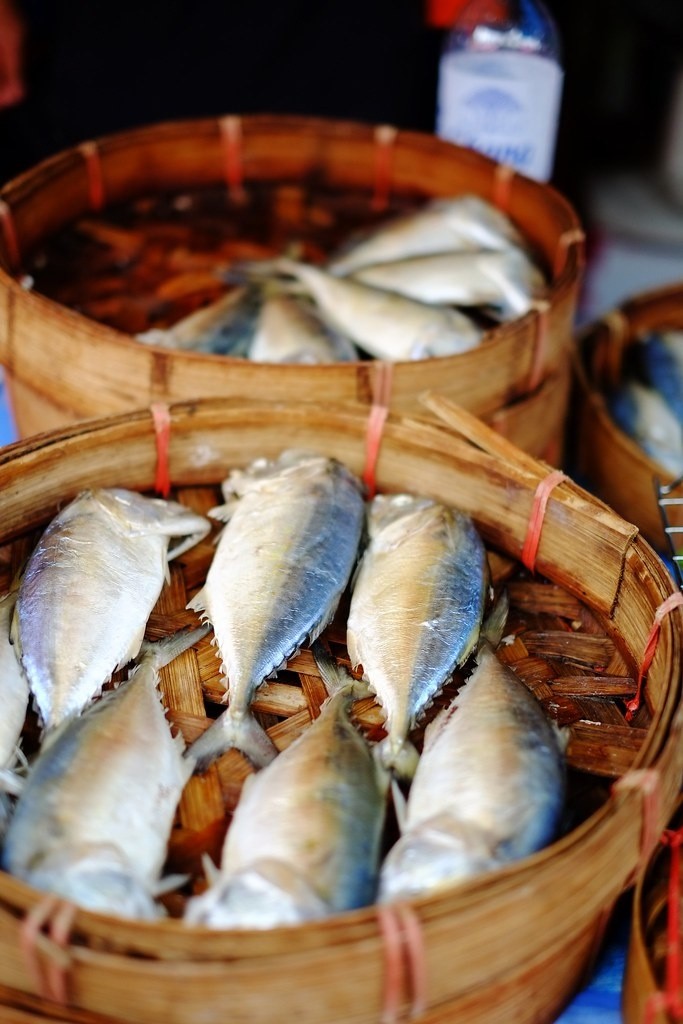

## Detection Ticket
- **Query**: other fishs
[0,457,561,931]
[133,194,544,363]
[607,321,682,468]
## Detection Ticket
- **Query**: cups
[438,51,563,179]
